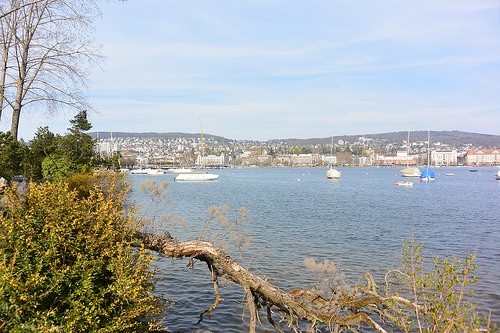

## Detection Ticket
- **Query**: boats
[393,181,413,186]
[325,164,341,179]
[493,171,500,180]
[121,168,193,176]
[468,169,479,173]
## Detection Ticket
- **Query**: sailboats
[419,128,435,183]
[401,123,422,177]
[174,129,219,182]
